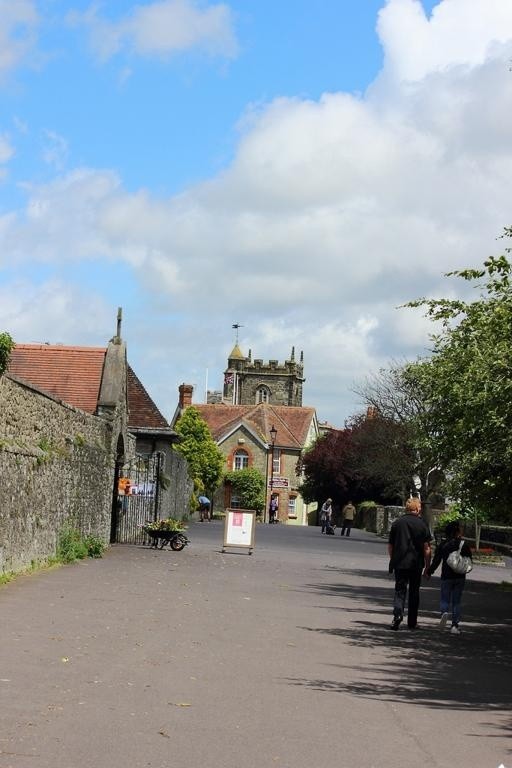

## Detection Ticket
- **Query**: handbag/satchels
[448,540,473,574]
[327,526,335,534]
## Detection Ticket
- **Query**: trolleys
[143,519,190,550]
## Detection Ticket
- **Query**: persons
[388,495,431,630]
[197,495,212,522]
[269,494,277,522]
[318,497,333,534]
[425,521,473,635]
[340,499,357,536]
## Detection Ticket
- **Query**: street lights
[270,424,277,524]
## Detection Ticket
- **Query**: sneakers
[451,626,460,634]
[439,612,448,626]
[391,615,402,629]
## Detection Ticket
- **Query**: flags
[224,374,234,385]
